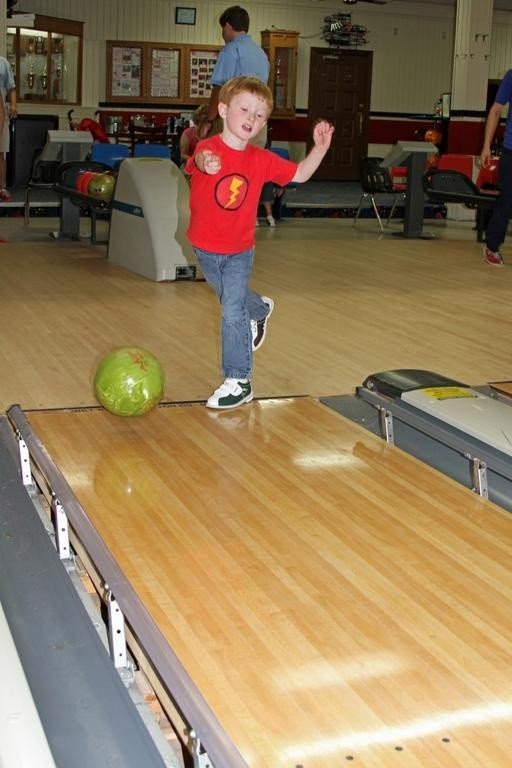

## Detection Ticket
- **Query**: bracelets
[204,117,214,124]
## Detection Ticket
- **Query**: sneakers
[206,377,253,408]
[483,248,504,265]
[266,216,275,226]
[250,295,274,351]
[0,189,13,202]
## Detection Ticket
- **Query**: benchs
[89,143,297,223]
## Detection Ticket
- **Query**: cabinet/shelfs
[6,9,87,107]
[258,29,301,122]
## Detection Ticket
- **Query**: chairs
[350,156,406,234]
[128,119,169,157]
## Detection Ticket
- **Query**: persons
[0,56,17,203]
[480,68,511,267]
[179,103,217,188]
[184,75,334,410]
[197,5,270,150]
[254,181,276,227]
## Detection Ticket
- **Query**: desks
[105,127,178,158]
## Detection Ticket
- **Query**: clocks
[175,7,197,25]
